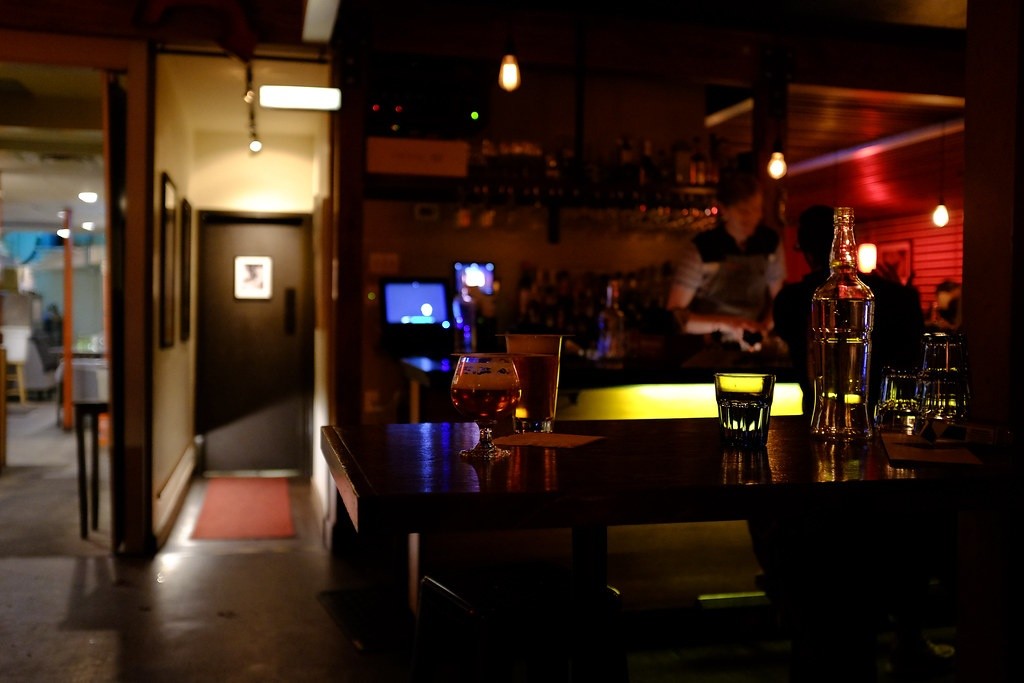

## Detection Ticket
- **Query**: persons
[44,304,64,346]
[773,205,928,413]
[667,174,784,349]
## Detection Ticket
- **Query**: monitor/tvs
[386,284,446,324]
[455,261,495,296]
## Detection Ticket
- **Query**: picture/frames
[158,172,177,348]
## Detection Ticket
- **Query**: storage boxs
[368,137,482,179]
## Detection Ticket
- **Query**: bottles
[598,279,624,359]
[810,207,876,439]
[516,260,670,326]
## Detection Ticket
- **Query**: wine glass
[449,354,522,459]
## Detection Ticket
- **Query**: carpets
[190,476,296,540]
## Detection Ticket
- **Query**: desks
[71,400,110,540]
[320,412,1023,682]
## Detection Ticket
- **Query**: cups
[913,330,970,420]
[873,363,920,430]
[505,335,562,433]
[471,137,723,210]
[714,372,776,448]
